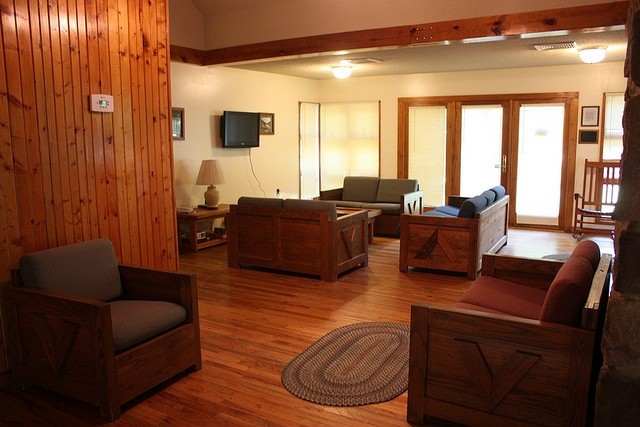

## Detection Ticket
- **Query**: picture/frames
[578,127,600,145]
[171,106,186,141]
[580,106,600,128]
[259,113,276,136]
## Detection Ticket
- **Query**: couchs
[226,196,369,283]
[399,185,511,282]
[408,239,612,427]
[312,176,425,239]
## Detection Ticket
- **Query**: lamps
[578,46,609,65]
[196,158,226,209]
[329,65,352,80]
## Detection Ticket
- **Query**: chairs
[571,159,623,241]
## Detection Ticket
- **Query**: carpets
[281,320,412,407]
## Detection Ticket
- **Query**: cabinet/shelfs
[176,203,230,253]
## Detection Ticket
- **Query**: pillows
[0,237,202,423]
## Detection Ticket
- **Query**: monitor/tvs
[220,110,261,150]
